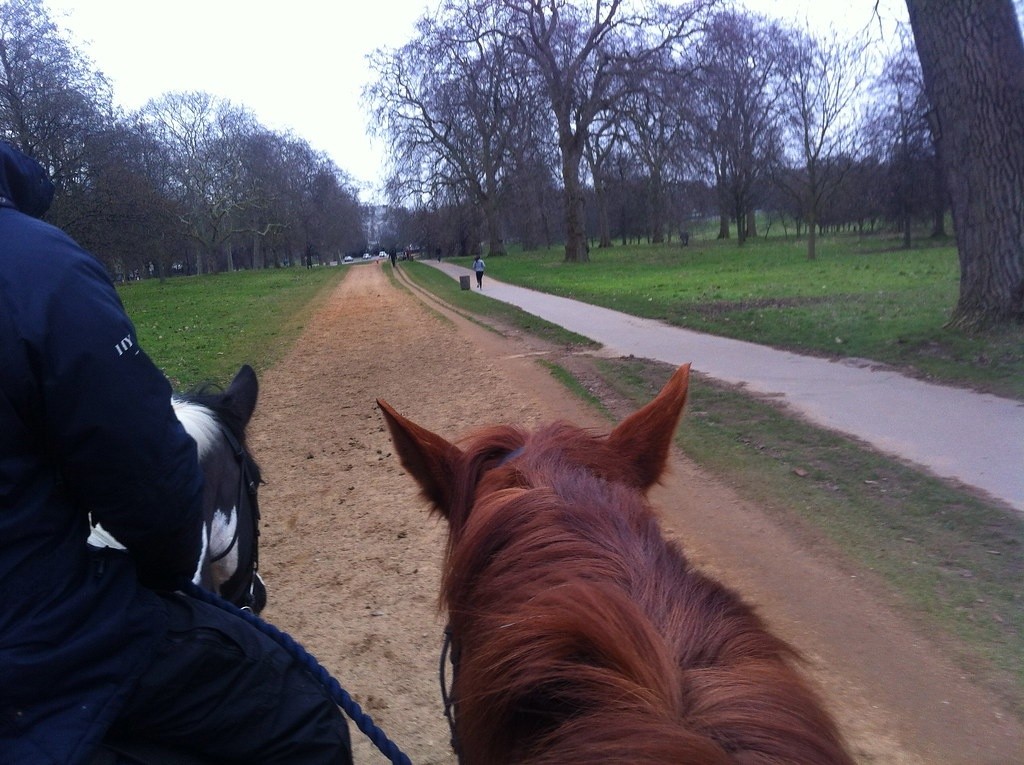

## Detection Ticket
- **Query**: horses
[375,356,866,765]
[88,362,269,763]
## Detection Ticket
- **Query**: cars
[379,251,387,257]
[344,256,354,262]
[363,253,372,259]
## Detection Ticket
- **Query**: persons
[435,245,440,263]
[0,137,354,765]
[388,249,397,268]
[473,256,486,290]
